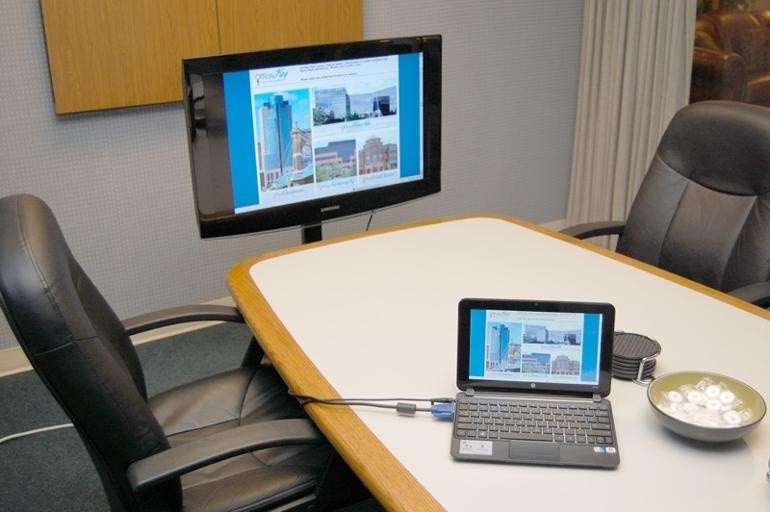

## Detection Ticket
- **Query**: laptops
[450,296,620,470]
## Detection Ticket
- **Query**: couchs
[690,6,770,100]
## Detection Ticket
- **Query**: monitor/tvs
[179,34,444,240]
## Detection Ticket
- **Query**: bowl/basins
[646,369,768,443]
